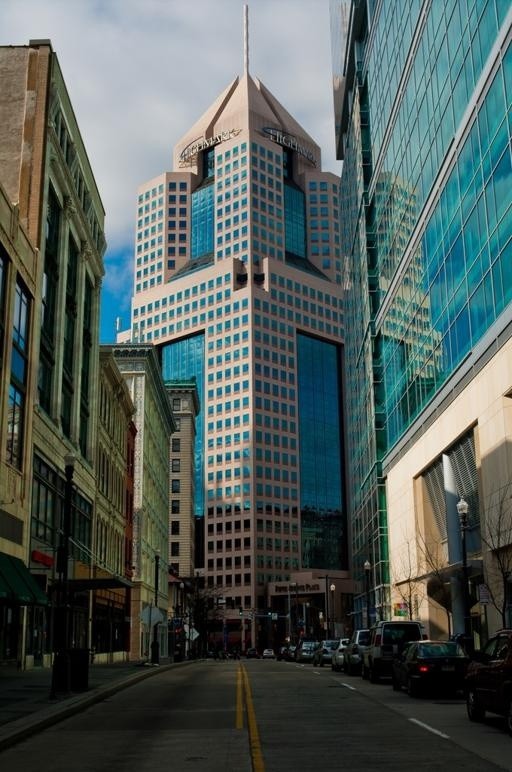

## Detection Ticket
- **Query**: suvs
[363,622,430,679]
[342,629,371,677]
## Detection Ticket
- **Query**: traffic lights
[239,607,244,616]
[299,627,304,636]
[249,610,254,621]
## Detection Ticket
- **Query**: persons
[294,638,304,663]
[312,639,324,666]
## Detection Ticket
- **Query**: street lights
[178,582,188,661]
[303,602,312,638]
[55,452,77,700]
[454,496,473,653]
[287,582,296,636]
[151,548,162,667]
[364,560,372,629]
[330,583,336,640]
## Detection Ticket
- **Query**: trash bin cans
[64,648,91,694]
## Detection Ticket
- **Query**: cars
[187,647,217,658]
[262,649,276,660]
[278,638,350,672]
[465,629,512,736]
[246,648,261,659]
[390,638,474,698]
